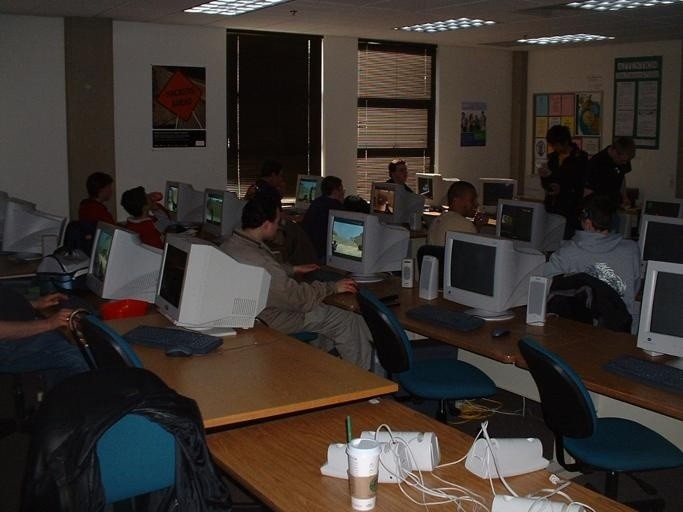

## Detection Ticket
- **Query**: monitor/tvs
[325,208,405,285]
[156,235,272,337]
[443,227,541,321]
[636,259,683,356]
[84,220,164,305]
[1,190,67,263]
[370,181,426,226]
[637,214,683,264]
[496,198,566,252]
[415,172,460,207]
[295,173,324,211]
[202,187,247,238]
[478,178,519,215]
[164,179,204,224]
[643,198,683,216]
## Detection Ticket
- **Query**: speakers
[464,436,550,480]
[360,430,441,472]
[419,251,437,300]
[320,441,413,484]
[524,276,548,327]
[490,492,585,512]
[396,255,417,289]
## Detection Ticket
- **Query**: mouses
[165,343,193,358]
[492,325,511,338]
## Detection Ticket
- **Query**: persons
[77,172,115,237]
[461,110,486,132]
[120,186,171,251]
[0,278,102,378]
[537,122,642,337]
[217,196,389,380]
[385,159,416,195]
[427,181,486,248]
[296,176,346,266]
[244,158,292,259]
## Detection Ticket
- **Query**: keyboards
[599,353,682,394]
[301,268,346,284]
[405,302,486,333]
[55,293,96,315]
[124,321,222,353]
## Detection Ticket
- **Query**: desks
[2,252,683,510]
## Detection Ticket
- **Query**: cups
[345,438,382,512]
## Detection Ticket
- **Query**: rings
[65,317,70,323]
